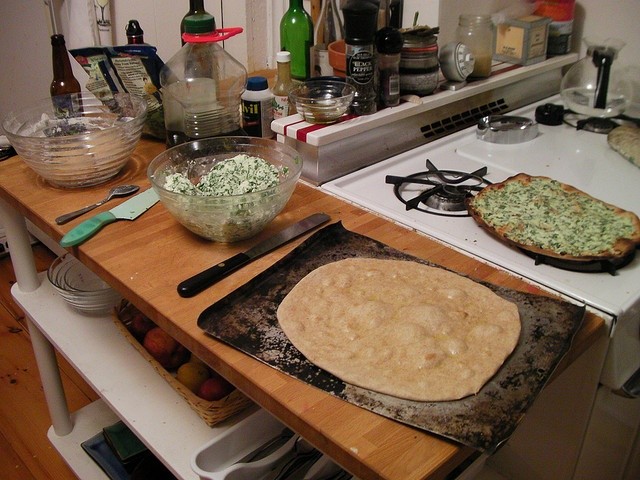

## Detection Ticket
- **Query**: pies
[377,27,404,109]
[278,257,521,401]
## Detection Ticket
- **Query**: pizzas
[469,172,636,263]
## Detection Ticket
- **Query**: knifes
[62,187,160,245]
[176,211,331,298]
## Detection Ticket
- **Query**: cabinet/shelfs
[0,137,605,480]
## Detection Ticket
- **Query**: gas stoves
[330,70,640,305]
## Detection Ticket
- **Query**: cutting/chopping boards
[193,218,605,456]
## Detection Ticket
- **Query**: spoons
[232,425,353,480]
[56,183,140,226]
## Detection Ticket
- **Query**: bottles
[278,2,313,80]
[456,15,493,79]
[343,5,378,116]
[48,34,84,116]
[271,51,297,121]
[239,77,277,139]
[120,19,150,48]
[178,3,218,59]
[314,2,344,76]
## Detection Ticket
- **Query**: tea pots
[561,37,632,121]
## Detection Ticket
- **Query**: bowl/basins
[153,132,304,243]
[45,251,121,317]
[328,39,363,73]
[292,81,356,124]
[1,92,162,186]
